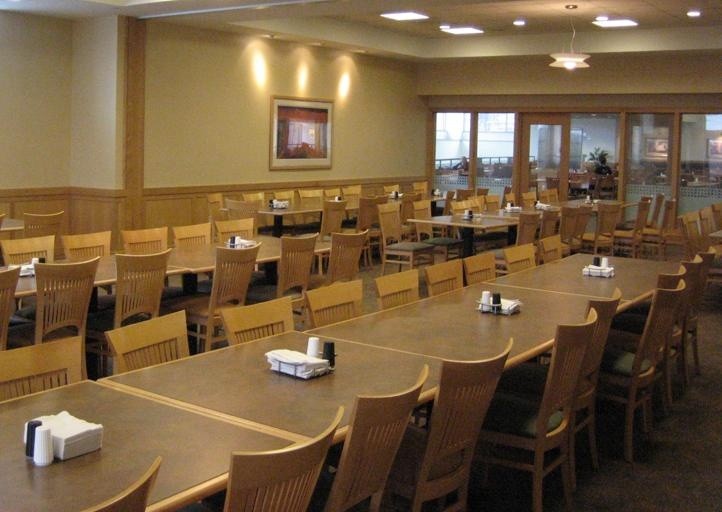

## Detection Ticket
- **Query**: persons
[588,157,612,196]
[460,157,469,170]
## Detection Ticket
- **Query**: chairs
[470,310,598,509]
[497,289,624,490]
[206,365,429,512]
[80,455,162,512]
[10,256,102,344]
[305,279,364,329]
[327,335,514,511]
[103,309,191,375]
[1,335,83,402]
[593,279,687,461]
[219,296,294,344]
[181,405,344,512]
[1,266,21,350]
[164,242,262,352]
[1,157,722,305]
[84,249,170,379]
[611,265,687,430]
[613,254,703,408]
[632,246,717,383]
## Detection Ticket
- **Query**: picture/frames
[268,93,333,172]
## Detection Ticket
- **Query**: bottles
[481,291,501,315]
[269,188,443,210]
[594,257,610,266]
[25,420,55,467]
[31,258,46,276]
[464,195,593,220]
[307,337,336,370]
[231,236,240,244]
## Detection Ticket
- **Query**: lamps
[548,3,591,71]
